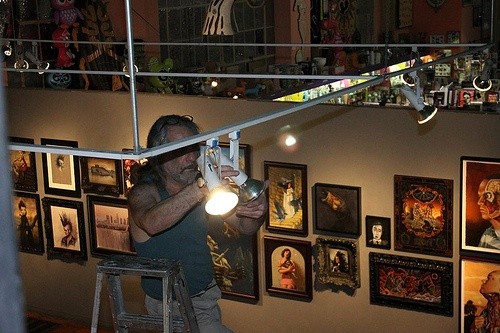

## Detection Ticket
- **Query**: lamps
[198,51,494,216]
[123,56,139,79]
[2,38,49,74]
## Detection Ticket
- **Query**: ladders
[91,255,200,333]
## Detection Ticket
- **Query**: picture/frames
[365,216,391,250]
[311,183,362,239]
[79,156,123,198]
[263,160,309,237]
[459,156,500,333]
[396,0,414,29]
[263,235,313,302]
[7,137,38,193]
[40,138,82,198]
[394,175,453,259]
[447,0,483,44]
[85,195,136,261]
[203,222,259,305]
[9,190,45,256]
[121,147,146,197]
[42,196,88,267]
[312,237,360,295]
[369,252,453,317]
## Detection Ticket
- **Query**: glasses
[160,115,193,131]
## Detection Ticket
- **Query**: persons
[128,115,266,333]
